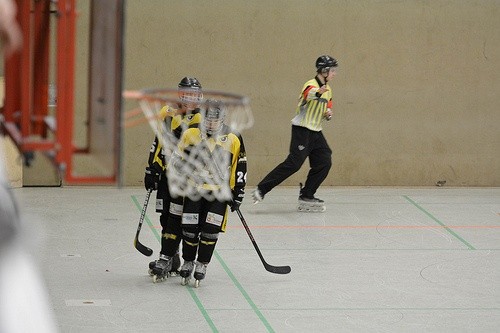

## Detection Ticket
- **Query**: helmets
[177,77,203,110]
[315,55,338,68]
[202,98,227,134]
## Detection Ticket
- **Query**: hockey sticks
[200,131,292,275]
[133,185,155,257]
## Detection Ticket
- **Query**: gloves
[229,189,244,212]
[144,167,159,191]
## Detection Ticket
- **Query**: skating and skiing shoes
[148,252,181,277]
[193,261,208,288]
[180,259,195,285]
[152,254,172,282]
[252,186,265,206]
[298,182,327,212]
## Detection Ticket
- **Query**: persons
[144,77,204,282]
[168,99,248,286]
[0,0,58,333]
[255,54,338,211]
